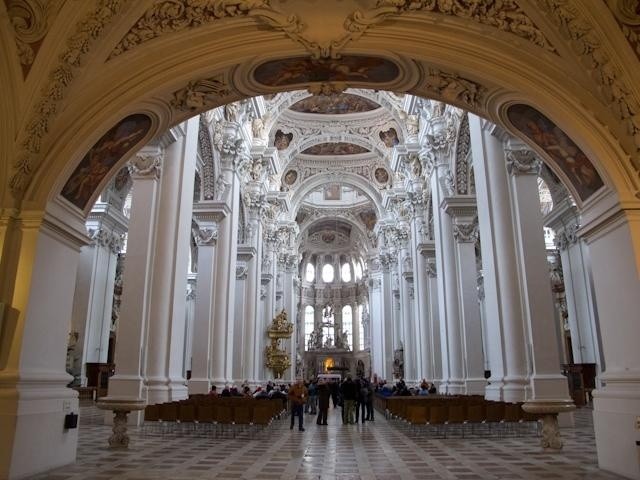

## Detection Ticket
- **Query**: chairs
[140,393,287,441]
[372,389,539,440]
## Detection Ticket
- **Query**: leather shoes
[342,418,374,425]
[316,422,328,425]
[299,427,305,431]
[290,426,293,429]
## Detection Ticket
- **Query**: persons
[393,359,402,379]
[357,360,364,373]
[209,372,436,432]
